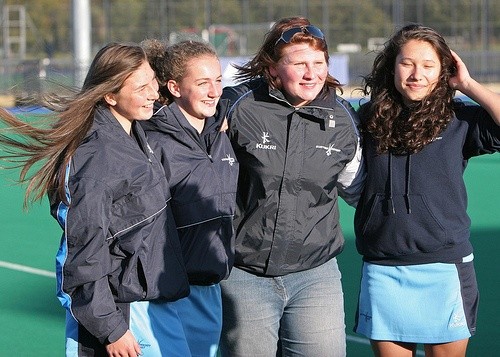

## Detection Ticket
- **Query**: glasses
[271,25,327,49]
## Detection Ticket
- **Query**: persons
[140,32,267,357]
[219,16,369,357]
[0,41,191,357]
[352,22,500,357]
